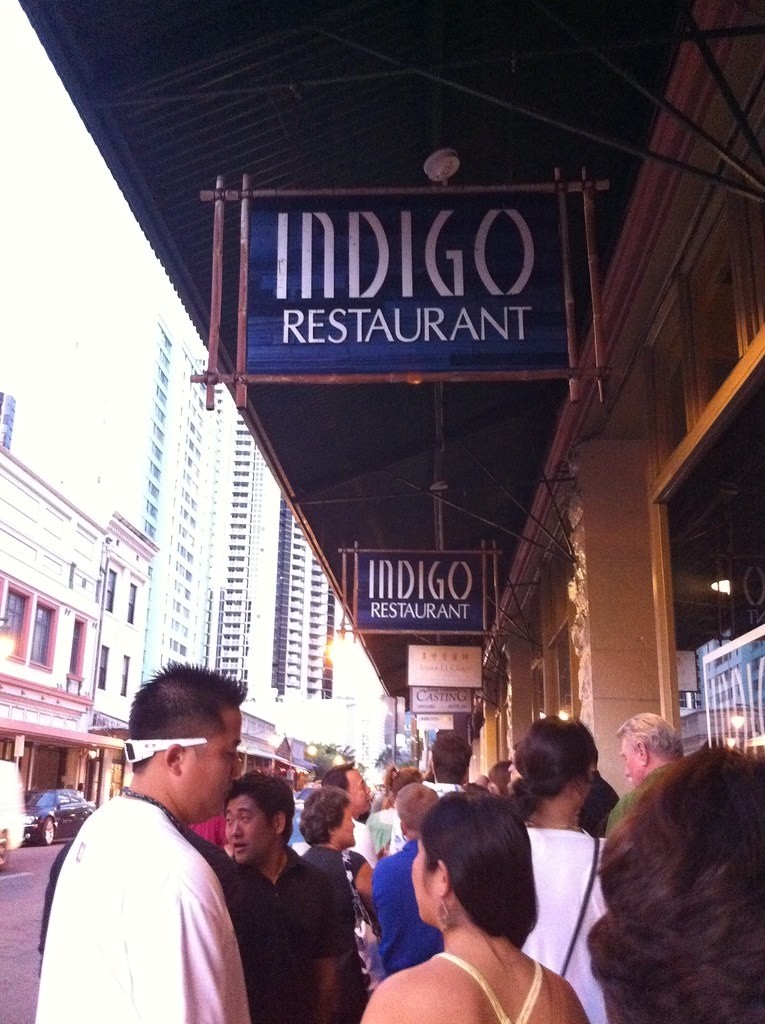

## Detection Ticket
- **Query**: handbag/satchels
[354,913,390,995]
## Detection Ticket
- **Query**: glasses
[122,737,208,764]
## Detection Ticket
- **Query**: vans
[1,760,26,871]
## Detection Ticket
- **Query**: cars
[23,788,96,846]
[289,787,318,857]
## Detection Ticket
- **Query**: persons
[359,791,590,1024]
[587,741,765,1024]
[35,661,252,1024]
[606,712,687,835]
[300,785,380,1023]
[321,762,389,998]
[365,729,514,975]
[225,769,339,1023]
[513,715,611,1022]
